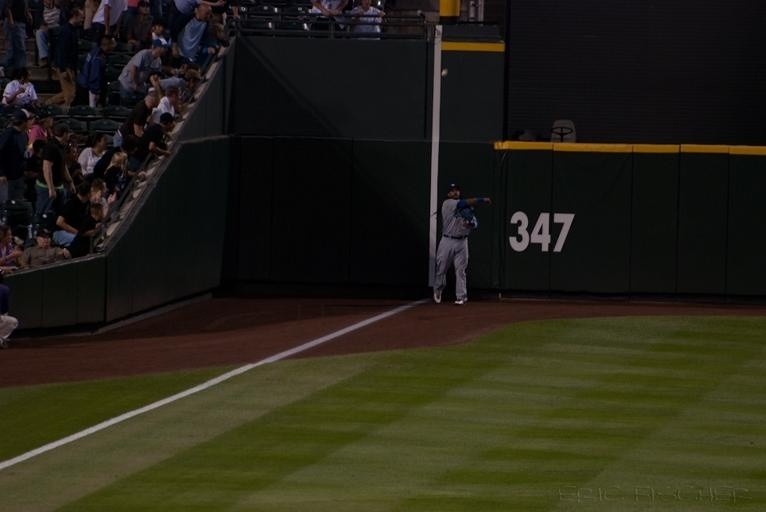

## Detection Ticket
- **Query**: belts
[443,233,468,240]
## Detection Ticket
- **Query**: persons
[221,1,384,40]
[2,1,230,274]
[433,182,492,305]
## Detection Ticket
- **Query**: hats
[13,106,52,123]
[151,36,173,49]
[36,228,53,239]
[446,182,462,194]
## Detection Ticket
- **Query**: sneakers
[433,288,442,304]
[452,294,469,305]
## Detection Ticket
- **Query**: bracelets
[476,197,484,205]
